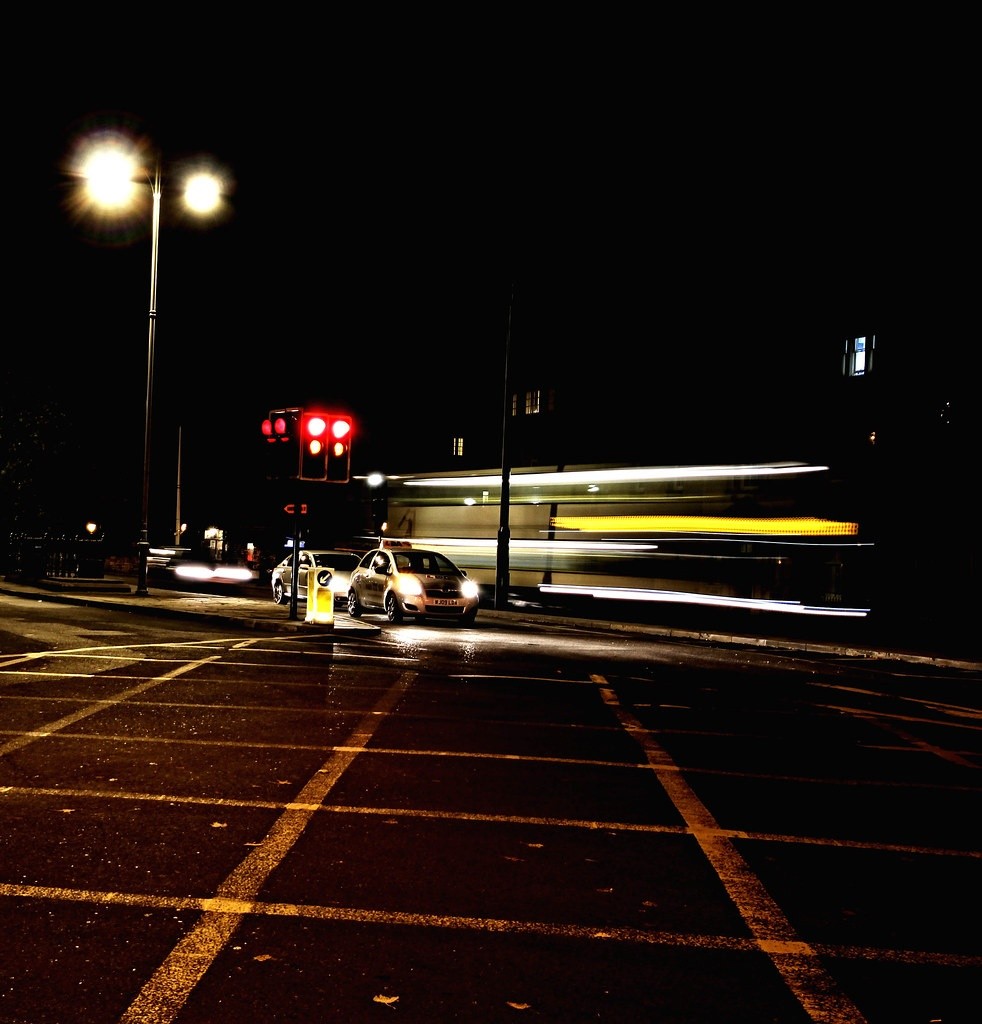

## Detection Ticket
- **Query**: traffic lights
[263,406,354,487]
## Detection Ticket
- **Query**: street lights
[88,140,215,604]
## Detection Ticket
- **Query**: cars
[344,544,479,622]
[269,546,359,609]
